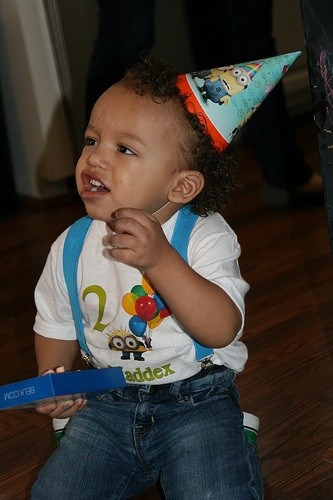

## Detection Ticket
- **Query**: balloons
[121,272,171,337]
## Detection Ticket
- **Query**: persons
[84,0,326,210]
[2,51,303,500]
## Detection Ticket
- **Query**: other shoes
[288,187,325,212]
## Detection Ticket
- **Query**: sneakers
[52,417,70,447]
[242,412,260,448]
